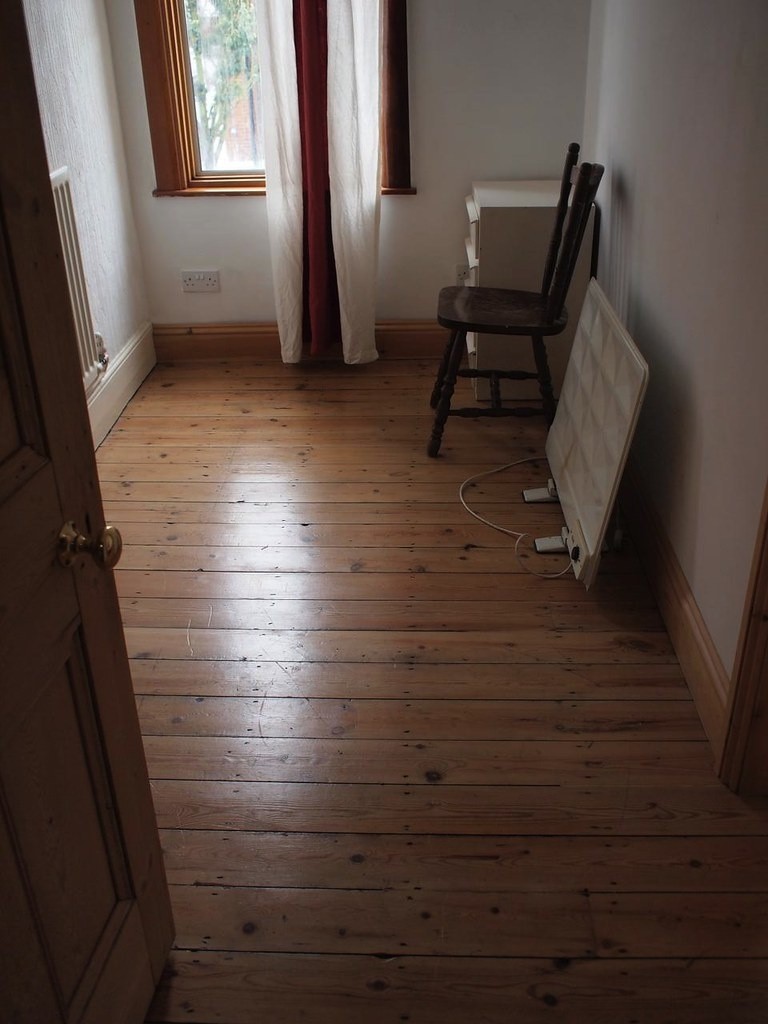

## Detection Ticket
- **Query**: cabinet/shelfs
[455,180,594,402]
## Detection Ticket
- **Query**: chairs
[423,142,605,457]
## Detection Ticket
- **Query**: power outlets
[566,520,589,582]
[180,269,221,293]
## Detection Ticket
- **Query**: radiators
[49,164,109,390]
